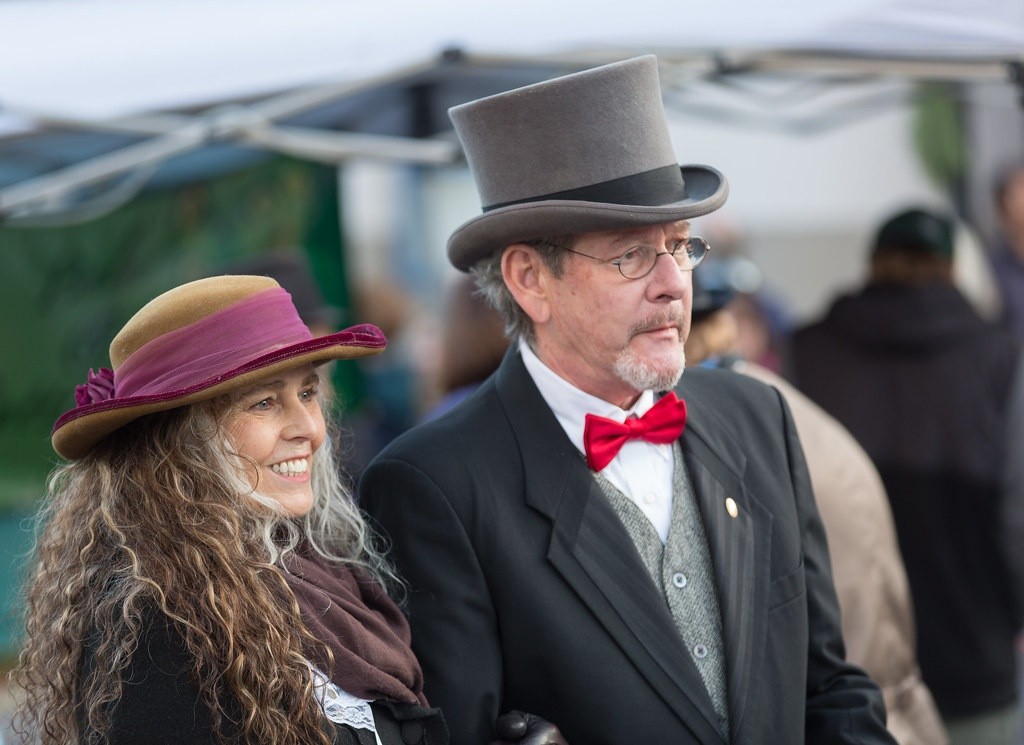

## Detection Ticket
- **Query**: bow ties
[584,392,687,473]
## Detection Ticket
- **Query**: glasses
[534,236,711,279]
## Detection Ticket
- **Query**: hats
[879,211,952,258]
[691,262,737,322]
[51,275,387,461]
[447,53,729,273]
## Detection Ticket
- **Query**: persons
[246,158,1024,745]
[356,52,902,745]
[12,275,567,744]
